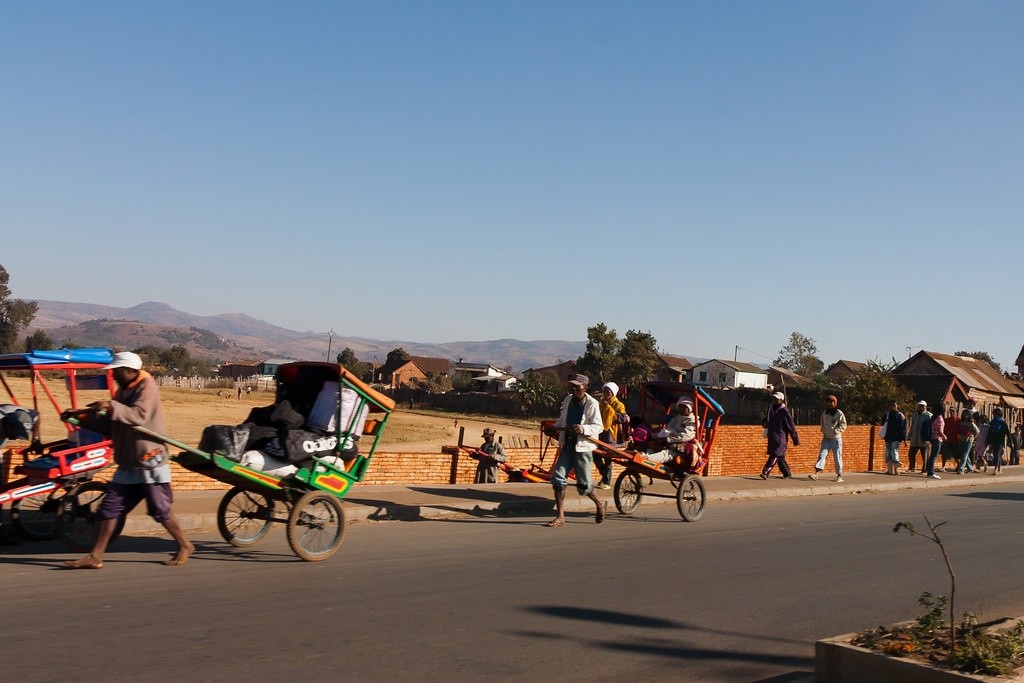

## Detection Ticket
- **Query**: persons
[409,398,413,410]
[546,373,608,527]
[928,402,947,480]
[64,352,196,569]
[592,381,627,489]
[760,391,800,480]
[198,383,252,400]
[808,396,847,482]
[907,400,933,475]
[880,400,908,476]
[639,396,700,464]
[936,398,1023,475]
[470,429,508,483]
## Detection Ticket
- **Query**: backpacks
[921,419,933,441]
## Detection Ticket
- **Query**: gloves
[653,437,670,447]
[653,427,663,433]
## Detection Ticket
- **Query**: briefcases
[309,382,370,439]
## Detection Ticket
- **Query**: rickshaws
[458,421,577,481]
[0,351,128,551]
[542,378,725,522]
[57,361,396,563]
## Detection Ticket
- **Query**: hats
[481,428,495,438]
[568,373,589,385]
[602,382,619,397]
[102,351,143,370]
[826,394,837,406]
[773,392,784,400]
[918,400,927,407]
[968,397,976,405]
[676,395,694,413]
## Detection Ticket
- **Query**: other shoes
[836,476,842,482]
[886,469,900,475]
[906,466,1003,479]
[781,474,791,479]
[808,474,817,481]
[760,473,768,480]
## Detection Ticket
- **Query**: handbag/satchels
[878,412,889,438]
[241,400,359,463]
[197,425,250,461]
[763,428,769,438]
